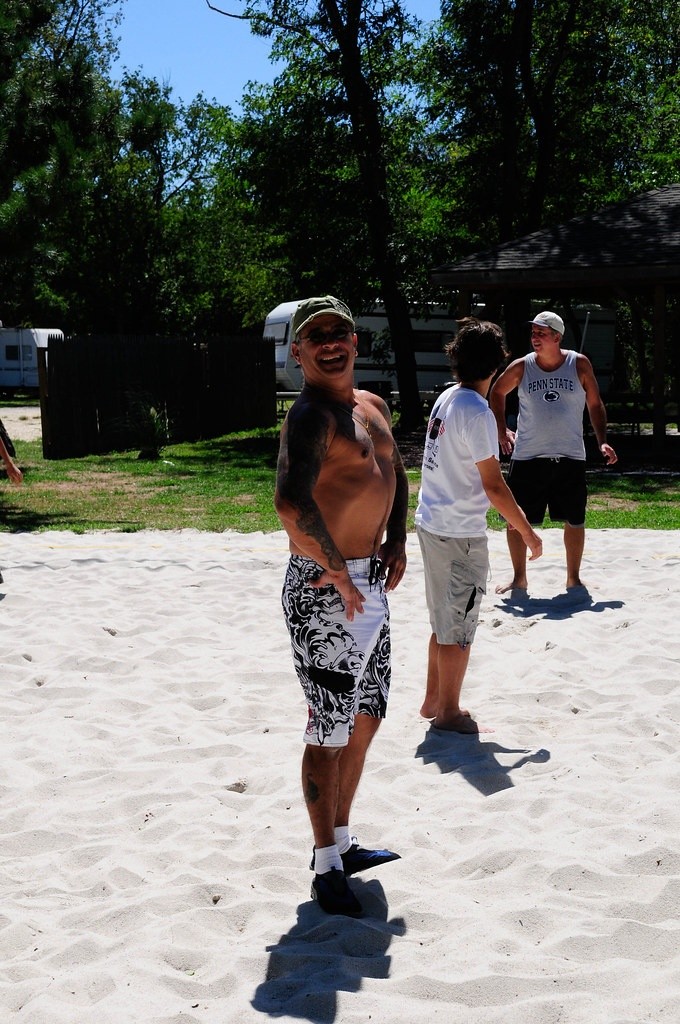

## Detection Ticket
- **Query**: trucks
[0,329,63,390]
[262,299,629,412]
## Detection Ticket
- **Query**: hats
[292,296,355,336]
[529,311,565,335]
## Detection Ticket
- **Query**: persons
[415,323,543,735]
[0,436,23,584]
[490,312,617,594]
[275,295,408,918]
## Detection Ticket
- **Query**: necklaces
[305,386,372,438]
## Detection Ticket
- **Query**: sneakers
[312,866,367,919]
[341,837,402,873]
